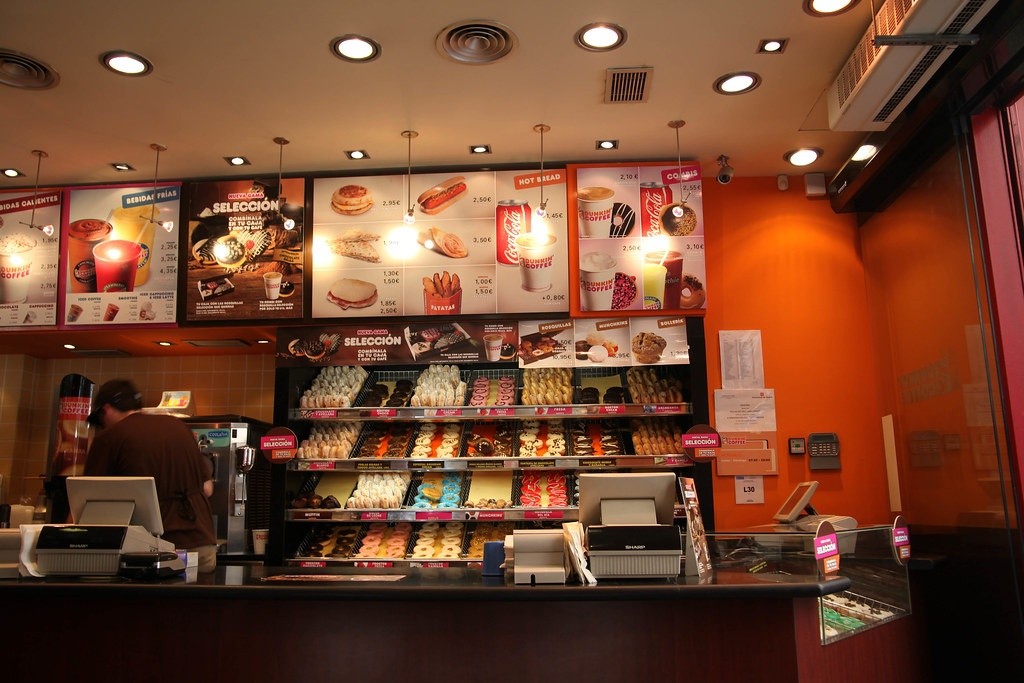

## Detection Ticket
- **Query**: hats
[85,379,142,427]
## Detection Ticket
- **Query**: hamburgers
[327,279,379,309]
[330,184,373,215]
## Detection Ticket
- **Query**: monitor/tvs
[66,477,164,537]
[578,473,676,526]
[774,480,818,522]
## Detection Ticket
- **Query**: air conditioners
[827,0,1000,132]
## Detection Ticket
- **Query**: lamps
[668,120,689,219]
[30,149,54,236]
[274,137,295,230]
[532,124,552,218]
[401,130,419,223]
[150,143,173,232]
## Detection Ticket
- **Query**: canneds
[640,181,673,236]
[496,200,532,266]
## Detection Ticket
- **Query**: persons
[66,378,216,565]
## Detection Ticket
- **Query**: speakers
[805,172,827,197]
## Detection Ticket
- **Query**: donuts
[303,521,508,558]
[356,419,622,458]
[410,470,571,510]
[500,343,516,359]
[521,336,556,356]
[609,201,705,309]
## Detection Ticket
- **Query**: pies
[326,231,382,264]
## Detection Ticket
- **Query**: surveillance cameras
[717,166,733,184]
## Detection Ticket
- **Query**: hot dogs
[418,176,467,216]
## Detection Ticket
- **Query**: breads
[631,416,686,455]
[288,338,326,359]
[191,179,298,269]
[418,227,468,258]
[574,332,667,363]
[292,471,410,509]
[298,365,684,409]
[296,417,364,457]
[818,593,892,640]
[409,323,464,354]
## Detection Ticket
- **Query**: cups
[580,260,619,312]
[515,232,558,293]
[68,218,113,293]
[0,240,38,306]
[483,542,504,576]
[483,334,503,362]
[112,205,160,287]
[67,304,83,322]
[263,272,283,301]
[92,239,143,294]
[577,186,616,240]
[251,528,269,555]
[23,311,36,324]
[103,303,120,321]
[642,250,684,310]
